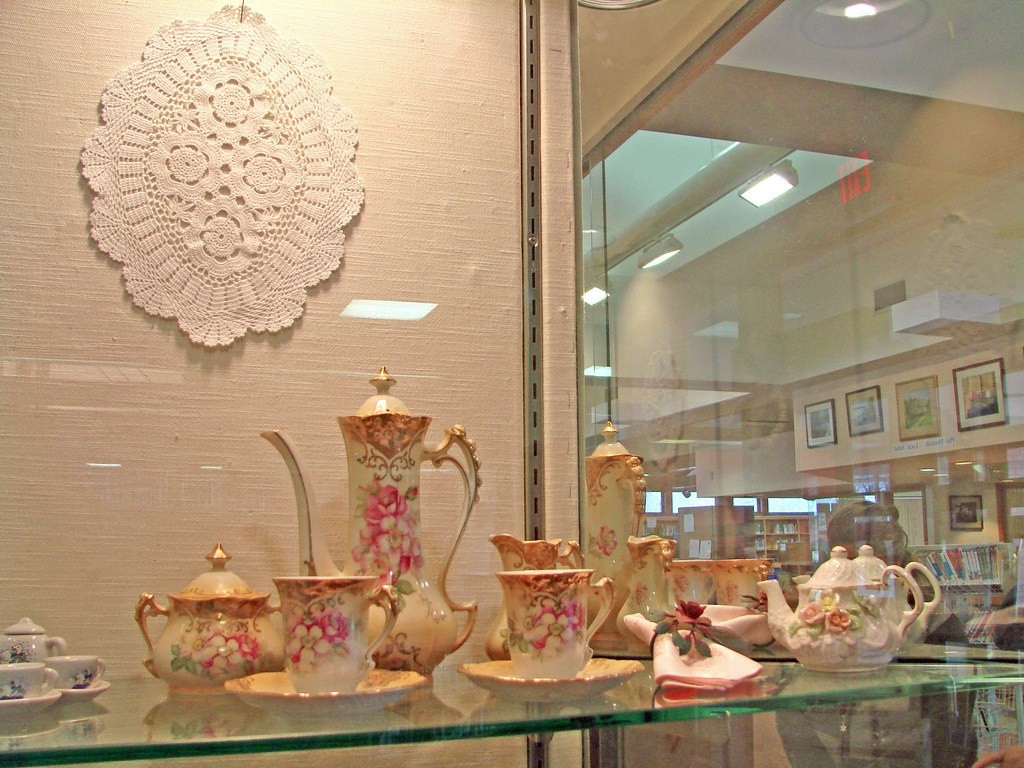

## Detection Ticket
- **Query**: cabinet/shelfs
[909,544,1016,619]
[751,514,813,576]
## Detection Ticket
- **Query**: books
[755,521,799,559]
[913,542,1018,647]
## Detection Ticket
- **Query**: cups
[618,535,677,642]
[665,559,716,606]
[43,655,105,688]
[1,663,58,700]
[710,559,771,616]
[484,533,585,660]
[495,568,616,681]
[273,574,400,693]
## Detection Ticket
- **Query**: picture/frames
[951,357,1009,432]
[802,399,839,448]
[844,384,884,437]
[895,374,942,442]
[947,493,983,530]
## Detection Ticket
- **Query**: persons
[972,745,1024,768]
[774,500,979,768]
[993,580,1024,653]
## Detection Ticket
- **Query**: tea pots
[756,546,924,673]
[261,367,483,674]
[584,420,647,634]
[133,543,289,698]
[791,545,942,652]
[0,617,68,664]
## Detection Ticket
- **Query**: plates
[0,713,58,738]
[456,658,645,703]
[0,685,64,717]
[225,668,426,714]
[49,704,112,722]
[56,681,111,703]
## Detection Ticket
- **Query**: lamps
[639,233,685,270]
[580,279,615,306]
[737,162,798,208]
